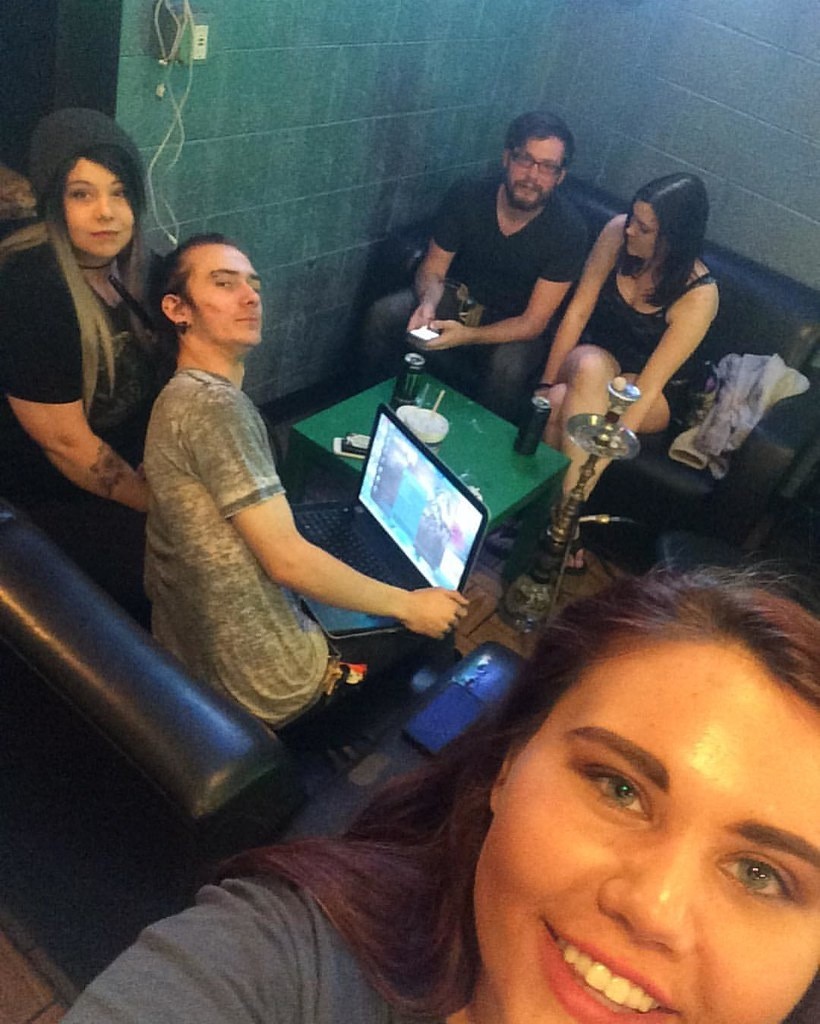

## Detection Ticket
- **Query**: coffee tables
[283,372,572,585]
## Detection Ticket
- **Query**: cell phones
[332,436,367,459]
[408,327,439,342]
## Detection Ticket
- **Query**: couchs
[348,167,820,575]
[0,498,528,859]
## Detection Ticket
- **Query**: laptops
[288,402,491,638]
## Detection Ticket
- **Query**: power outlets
[191,24,210,60]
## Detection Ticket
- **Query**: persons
[142,233,470,739]
[362,111,587,425]
[61,566,819,1024]
[0,108,176,518]
[532,173,719,574]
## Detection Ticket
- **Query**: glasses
[509,145,564,175]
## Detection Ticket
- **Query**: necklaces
[79,257,115,269]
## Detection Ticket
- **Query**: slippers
[483,520,521,559]
[560,539,587,576]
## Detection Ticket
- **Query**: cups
[396,405,450,456]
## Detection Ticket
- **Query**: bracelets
[537,383,552,388]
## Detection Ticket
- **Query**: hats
[26,108,144,191]
